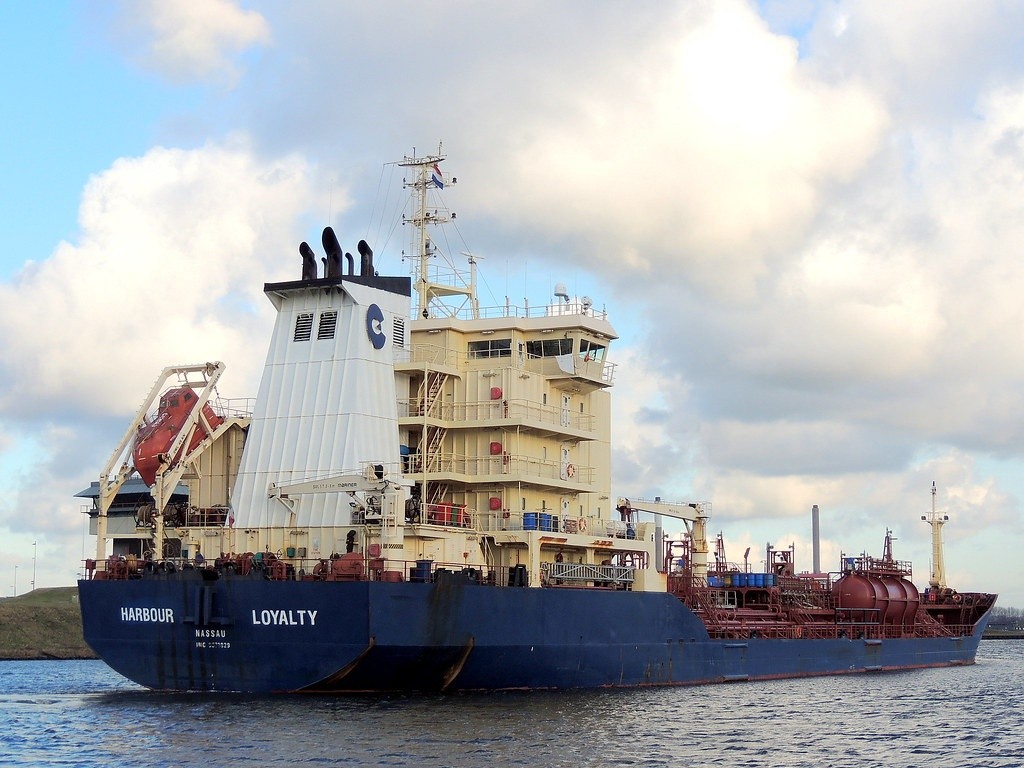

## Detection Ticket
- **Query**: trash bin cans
[415,559,434,583]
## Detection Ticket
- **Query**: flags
[432,164,444,190]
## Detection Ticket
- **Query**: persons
[194,549,206,567]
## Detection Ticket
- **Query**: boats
[130,385,224,489]
[982,621,1024,640]
[69,141,1000,699]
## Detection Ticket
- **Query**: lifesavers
[144,561,157,575]
[159,561,170,575]
[272,561,287,580]
[567,464,575,478]
[205,509,221,526]
[579,518,588,532]
[928,592,936,603]
[953,594,962,603]
[312,562,324,575]
